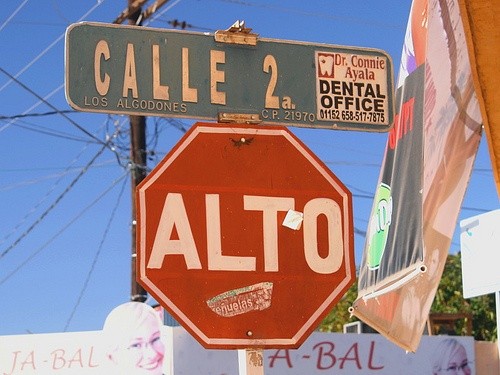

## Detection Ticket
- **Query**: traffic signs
[64,21,395,132]
[135,122,355,349]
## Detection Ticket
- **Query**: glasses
[447,361,473,372]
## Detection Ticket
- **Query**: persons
[432,340,471,374]
[101,301,167,374]
